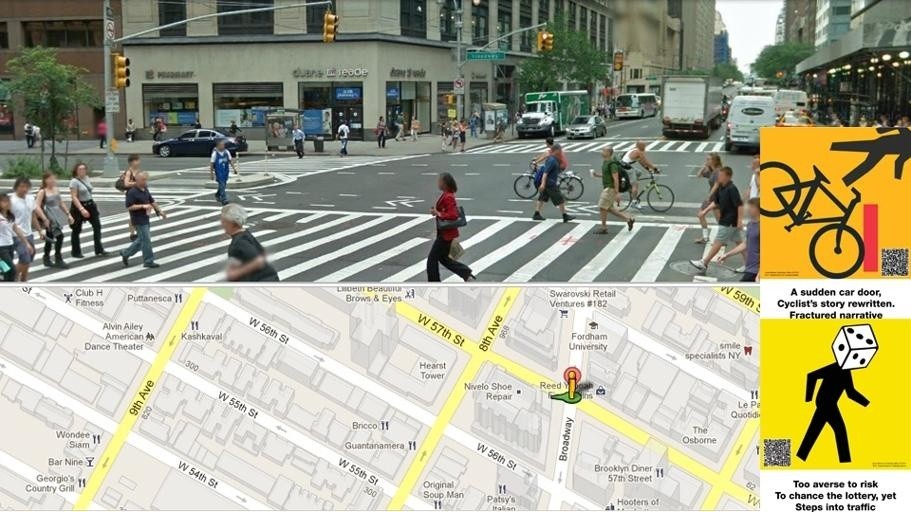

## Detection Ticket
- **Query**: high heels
[463,267,476,281]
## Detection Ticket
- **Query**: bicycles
[599,166,679,214]
[511,157,587,200]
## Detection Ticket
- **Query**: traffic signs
[465,50,507,61]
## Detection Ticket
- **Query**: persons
[0,160,110,282]
[425,171,478,282]
[596,101,615,119]
[118,168,167,270]
[20,118,39,147]
[393,111,408,141]
[440,111,509,153]
[336,119,350,157]
[116,153,141,238]
[828,111,911,128]
[229,119,241,135]
[409,115,420,142]
[531,135,659,234]
[688,152,761,282]
[124,118,136,140]
[290,124,306,159]
[219,202,278,281]
[374,116,389,149]
[193,118,201,128]
[322,110,331,133]
[208,139,237,204]
[148,115,166,141]
[96,117,108,149]
[268,122,289,138]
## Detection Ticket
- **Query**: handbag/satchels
[534,165,546,190]
[115,169,134,192]
[436,206,467,231]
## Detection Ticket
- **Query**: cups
[590,169,595,179]
[146,206,151,216]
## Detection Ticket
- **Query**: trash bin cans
[314,137,324,152]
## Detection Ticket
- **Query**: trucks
[659,69,816,157]
[516,90,589,138]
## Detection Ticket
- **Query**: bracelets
[141,204,144,209]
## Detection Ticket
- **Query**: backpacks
[340,126,347,136]
[608,162,631,194]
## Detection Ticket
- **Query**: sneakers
[95,248,110,255]
[736,265,745,272]
[627,216,636,232]
[215,192,221,202]
[55,258,69,269]
[563,212,576,223]
[690,258,707,272]
[222,199,229,206]
[43,255,54,267]
[120,251,129,267]
[592,227,608,235]
[695,236,710,245]
[71,251,84,258]
[533,214,546,221]
[144,262,160,268]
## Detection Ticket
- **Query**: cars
[152,127,251,160]
[565,113,610,138]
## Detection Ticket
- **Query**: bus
[612,92,657,118]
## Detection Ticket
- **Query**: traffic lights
[537,32,546,51]
[545,31,554,52]
[324,11,341,44]
[114,53,133,89]
[443,95,453,105]
